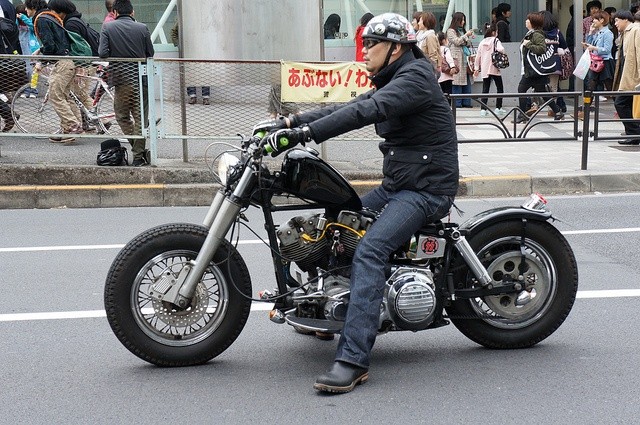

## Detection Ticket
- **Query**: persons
[353,13,374,61]
[0,16,29,132]
[0,0,16,23]
[495,3,513,42]
[412,12,423,33]
[511,12,564,123]
[25,0,76,144]
[474,23,506,117]
[523,11,573,115]
[418,12,451,111]
[93,0,161,128]
[566,0,640,146]
[447,12,473,108]
[98,0,154,168]
[251,11,459,392]
[324,13,341,39]
[16,1,41,101]
[47,0,112,134]
[170,19,210,105]
[434,15,445,37]
[485,7,497,36]
[437,31,457,106]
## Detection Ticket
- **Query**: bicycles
[9,61,163,143]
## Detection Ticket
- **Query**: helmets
[361,13,417,43]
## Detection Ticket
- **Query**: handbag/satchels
[527,44,556,78]
[573,49,590,80]
[97,147,128,165]
[1,59,28,92]
[442,59,460,74]
[467,55,481,72]
[67,31,92,62]
[560,52,574,80]
[632,95,640,119]
[590,52,604,72]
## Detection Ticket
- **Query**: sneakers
[593,96,607,101]
[548,110,554,116]
[570,111,584,120]
[143,149,150,164]
[481,109,487,117]
[562,106,566,112]
[49,126,84,143]
[101,122,112,135]
[2,114,19,132]
[525,105,539,116]
[456,106,461,108]
[494,109,506,115]
[462,105,473,107]
[132,157,145,167]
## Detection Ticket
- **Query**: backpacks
[491,51,509,69]
[67,17,100,56]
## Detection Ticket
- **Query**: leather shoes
[618,140,639,145]
[313,361,370,393]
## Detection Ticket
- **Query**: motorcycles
[104,112,579,368]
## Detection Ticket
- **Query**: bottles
[30,66,38,88]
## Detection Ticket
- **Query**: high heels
[554,112,564,120]
[511,113,526,123]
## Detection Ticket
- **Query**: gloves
[252,117,288,135]
[258,129,306,157]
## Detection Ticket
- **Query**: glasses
[363,39,383,48]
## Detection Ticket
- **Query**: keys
[328,226,349,259]
